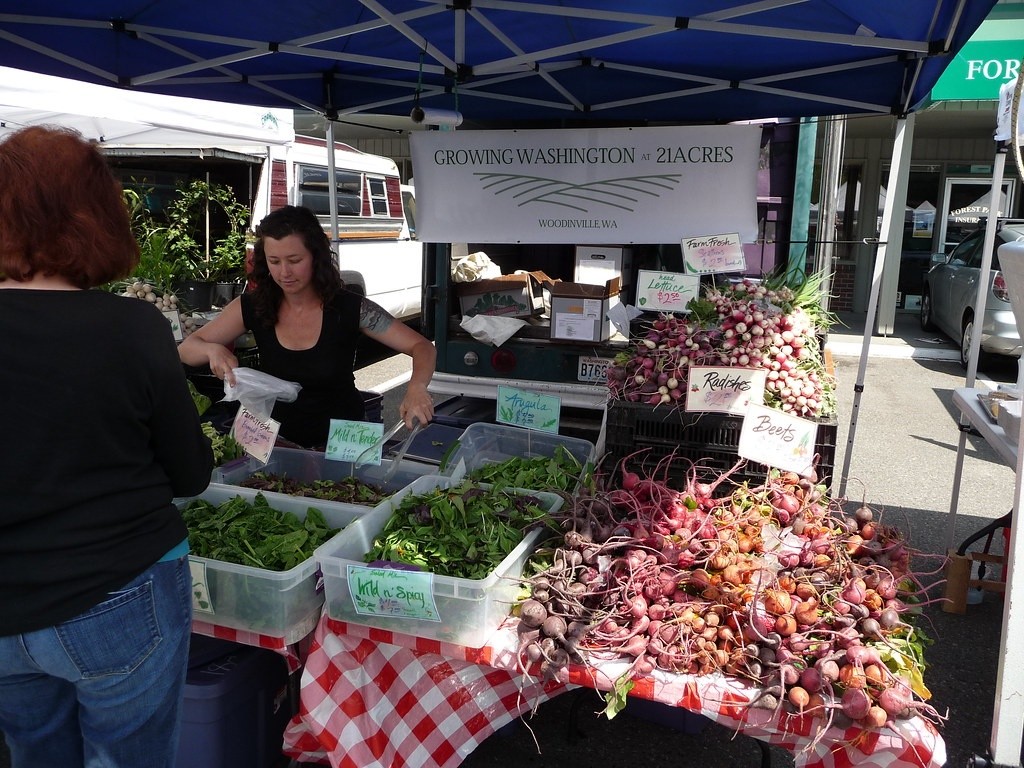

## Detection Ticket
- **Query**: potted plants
[207,229,248,306]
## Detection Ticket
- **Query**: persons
[177,206,437,450]
[1,126,215,768]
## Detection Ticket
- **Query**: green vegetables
[181,421,594,583]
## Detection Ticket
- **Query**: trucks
[420,241,713,414]
[89,132,468,350]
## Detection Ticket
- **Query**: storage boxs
[170,417,595,648]
[454,269,551,317]
[541,276,620,342]
[602,395,837,497]
[996,398,1021,445]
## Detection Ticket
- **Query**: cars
[919,225,1024,373]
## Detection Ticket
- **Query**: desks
[282,603,947,768]
[942,386,1018,612]
